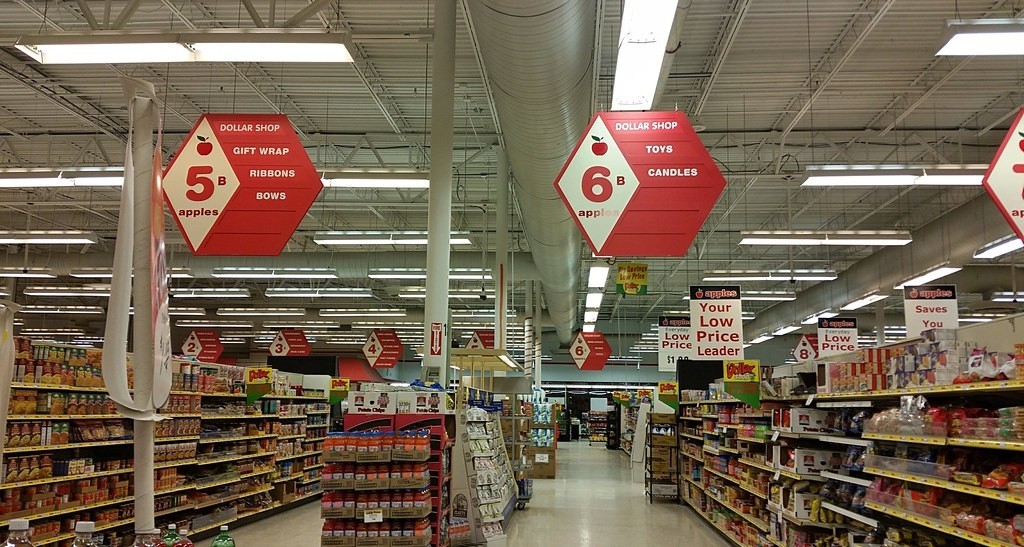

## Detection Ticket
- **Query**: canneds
[0,468,178,535]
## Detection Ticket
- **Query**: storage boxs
[320,535,356,547]
[389,473,431,490]
[321,449,356,462]
[645,412,676,424]
[645,457,668,472]
[356,535,391,547]
[770,406,838,433]
[319,478,354,490]
[647,434,676,445]
[390,529,433,546]
[0,0,1024,351]
[355,478,390,491]
[320,506,355,519]
[398,390,456,415]
[391,447,431,461]
[390,501,432,518]
[766,482,819,519]
[355,450,392,462]
[771,445,847,476]
[346,390,398,413]
[354,507,391,518]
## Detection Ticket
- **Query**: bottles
[130,523,194,547]
[0,519,35,547]
[323,429,431,537]
[210,526,235,547]
[71,521,98,547]
[449,520,471,537]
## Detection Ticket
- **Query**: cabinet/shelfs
[0,328,570,547]
[583,316,1024,547]
